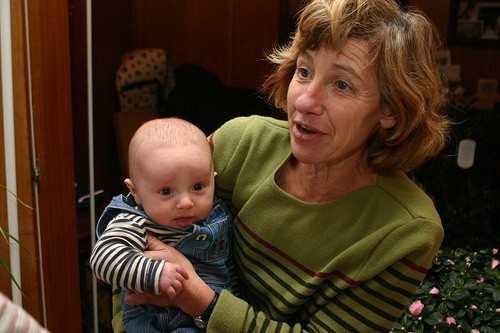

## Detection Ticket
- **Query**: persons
[111,0,450,332]
[88,117,243,333]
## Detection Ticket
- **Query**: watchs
[191,292,221,329]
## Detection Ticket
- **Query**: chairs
[114,105,159,180]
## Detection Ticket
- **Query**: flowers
[403,247,500,333]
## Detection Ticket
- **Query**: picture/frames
[447,0,500,50]
